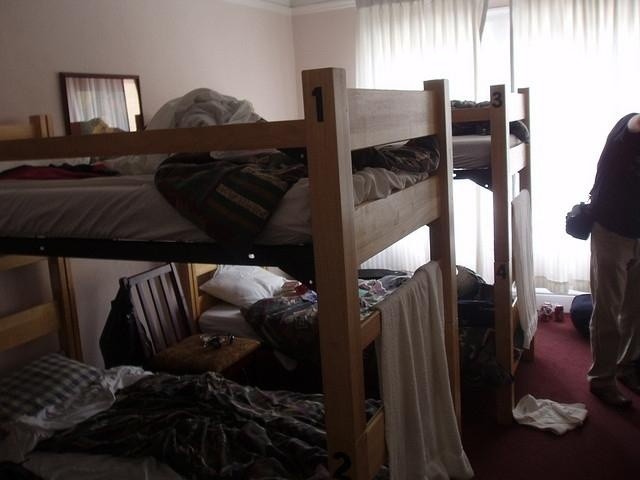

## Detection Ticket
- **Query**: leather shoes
[618,376,640,394]
[595,388,631,408]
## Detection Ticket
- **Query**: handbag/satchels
[99,286,155,368]
[565,202,592,240]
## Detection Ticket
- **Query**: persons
[586,112,640,409]
